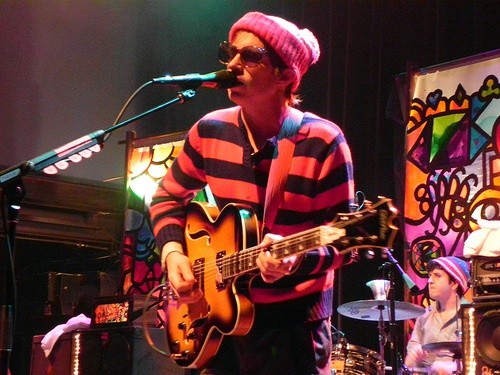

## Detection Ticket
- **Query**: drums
[330,342,386,375]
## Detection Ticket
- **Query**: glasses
[216,41,269,67]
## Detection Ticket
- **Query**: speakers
[30,326,134,374]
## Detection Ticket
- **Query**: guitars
[161,197,399,370]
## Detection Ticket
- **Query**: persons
[404,255,473,375]
[149,11,356,375]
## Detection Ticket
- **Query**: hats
[430,256,471,294]
[229,12,320,92]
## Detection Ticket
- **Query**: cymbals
[421,341,464,358]
[335,299,427,322]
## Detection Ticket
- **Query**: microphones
[152,70,236,88]
